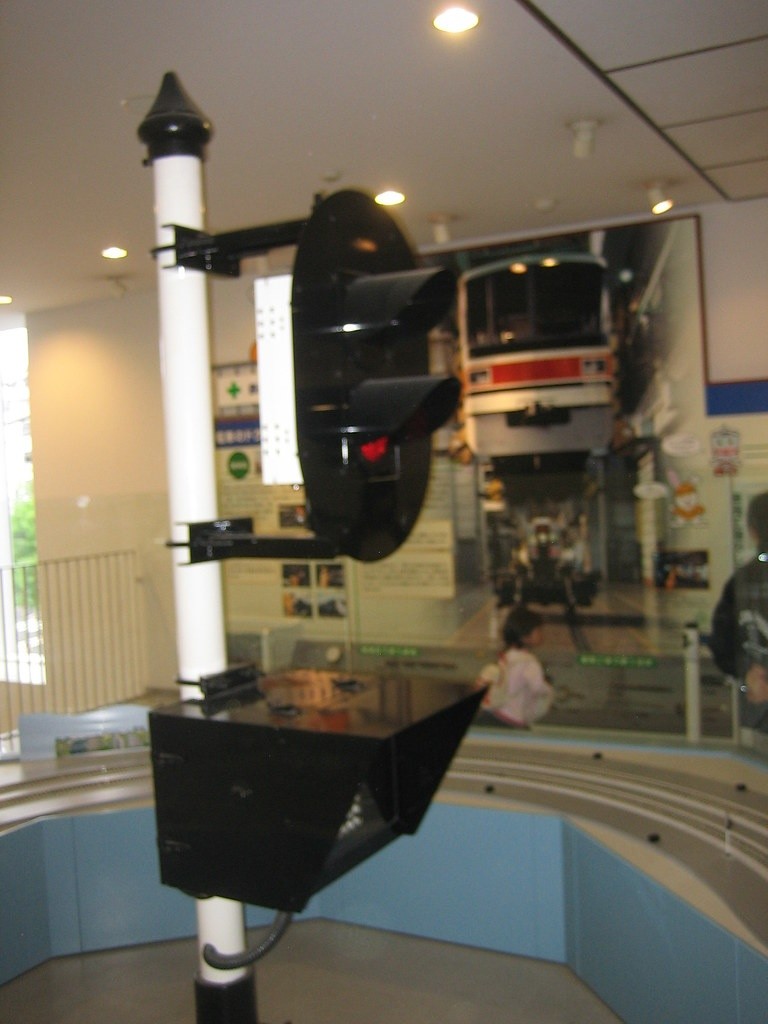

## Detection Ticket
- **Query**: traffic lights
[291,186,462,565]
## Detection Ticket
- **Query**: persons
[709,491,768,732]
[490,608,555,729]
[496,561,527,610]
